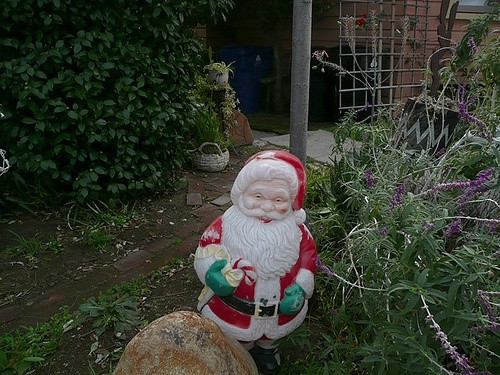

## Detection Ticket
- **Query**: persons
[193,150,318,371]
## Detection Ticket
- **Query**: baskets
[192,142,230,172]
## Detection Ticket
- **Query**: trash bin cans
[214,43,383,122]
